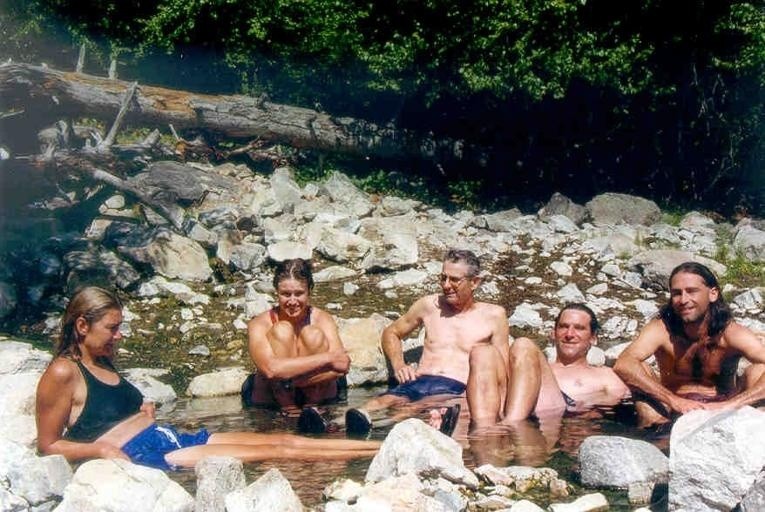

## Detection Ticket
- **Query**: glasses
[437,273,472,289]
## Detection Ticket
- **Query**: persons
[612,260,765,426]
[296,249,511,432]
[36,286,460,472]
[241,258,351,418]
[467,303,629,426]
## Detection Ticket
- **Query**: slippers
[344,407,373,434]
[439,404,461,437]
[296,407,327,434]
